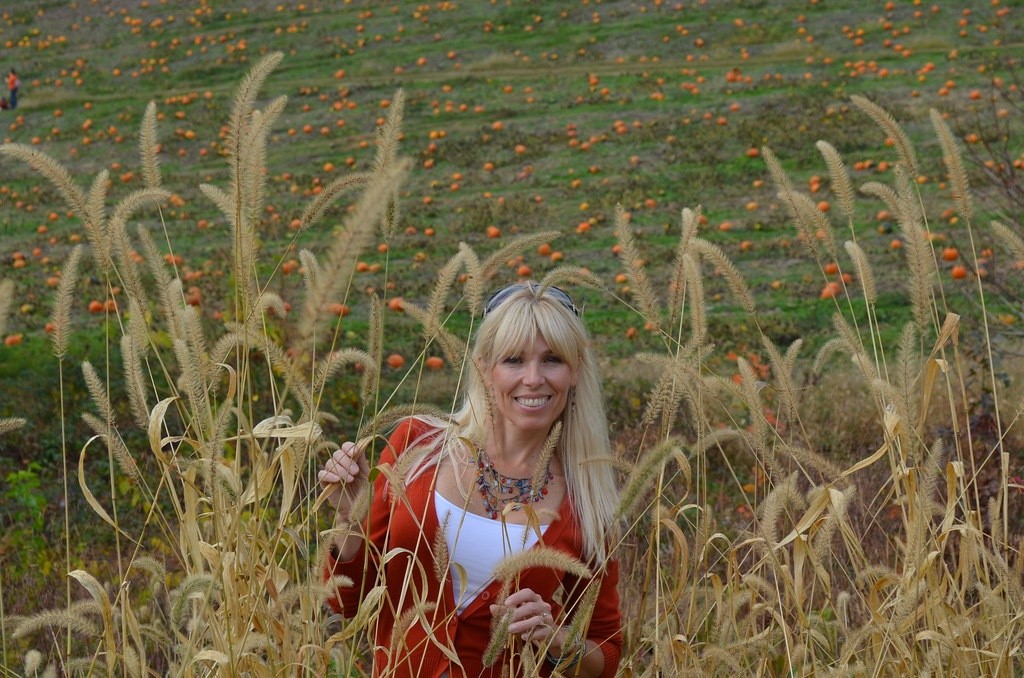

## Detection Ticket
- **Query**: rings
[542,612,548,624]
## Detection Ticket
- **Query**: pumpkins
[0,0,1024,519]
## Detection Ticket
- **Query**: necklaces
[472,444,552,520]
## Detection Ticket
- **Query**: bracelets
[545,625,588,669]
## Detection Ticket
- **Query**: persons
[4,67,20,107]
[319,282,624,678]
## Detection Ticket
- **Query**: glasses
[481,283,580,318]
